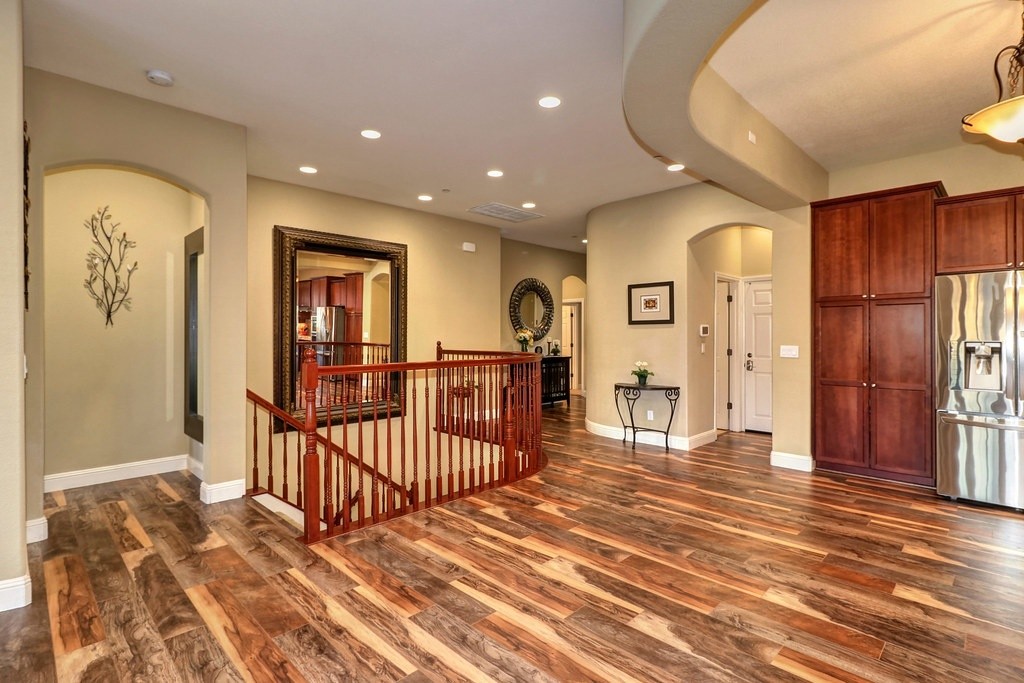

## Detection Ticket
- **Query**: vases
[637,375,647,384]
[521,344,529,352]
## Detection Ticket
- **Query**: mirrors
[272,224,408,435]
[509,278,554,341]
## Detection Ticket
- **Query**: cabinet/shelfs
[807,180,1024,490]
[298,272,362,381]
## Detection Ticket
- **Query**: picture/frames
[628,281,674,324]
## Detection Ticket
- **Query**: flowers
[513,329,533,346]
[631,361,654,377]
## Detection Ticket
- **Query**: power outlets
[462,242,475,252]
[647,410,653,420]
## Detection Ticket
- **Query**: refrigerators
[931,271,1024,516]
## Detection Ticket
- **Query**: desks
[614,383,680,450]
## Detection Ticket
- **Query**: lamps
[961,0,1024,143]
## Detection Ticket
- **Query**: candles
[553,340,560,345]
[547,337,551,342]
